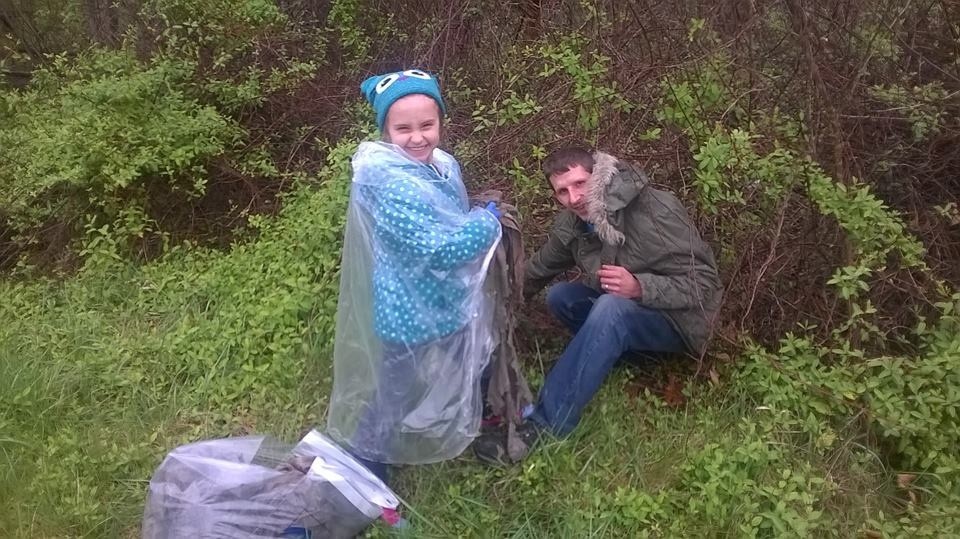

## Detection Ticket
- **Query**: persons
[476,137,727,467]
[350,69,534,481]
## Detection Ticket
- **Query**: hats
[359,69,446,136]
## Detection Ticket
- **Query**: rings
[606,284,608,290]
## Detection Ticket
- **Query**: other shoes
[473,402,534,427]
[472,425,541,470]
[378,507,412,530]
